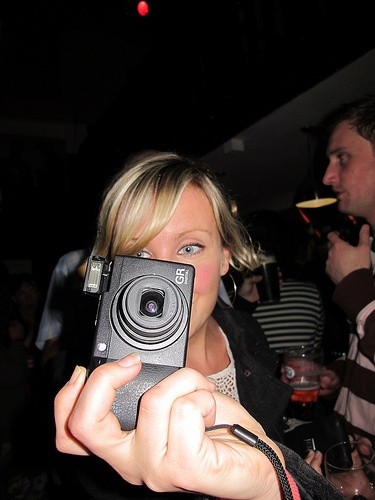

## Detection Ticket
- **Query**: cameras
[83,255,196,432]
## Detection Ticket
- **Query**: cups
[285,345,324,390]
[252,256,281,302]
[324,441,375,500]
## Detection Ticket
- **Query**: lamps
[292,124,340,209]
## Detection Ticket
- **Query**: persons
[0,242,375,500]
[19,149,289,500]
[287,101,375,476]
